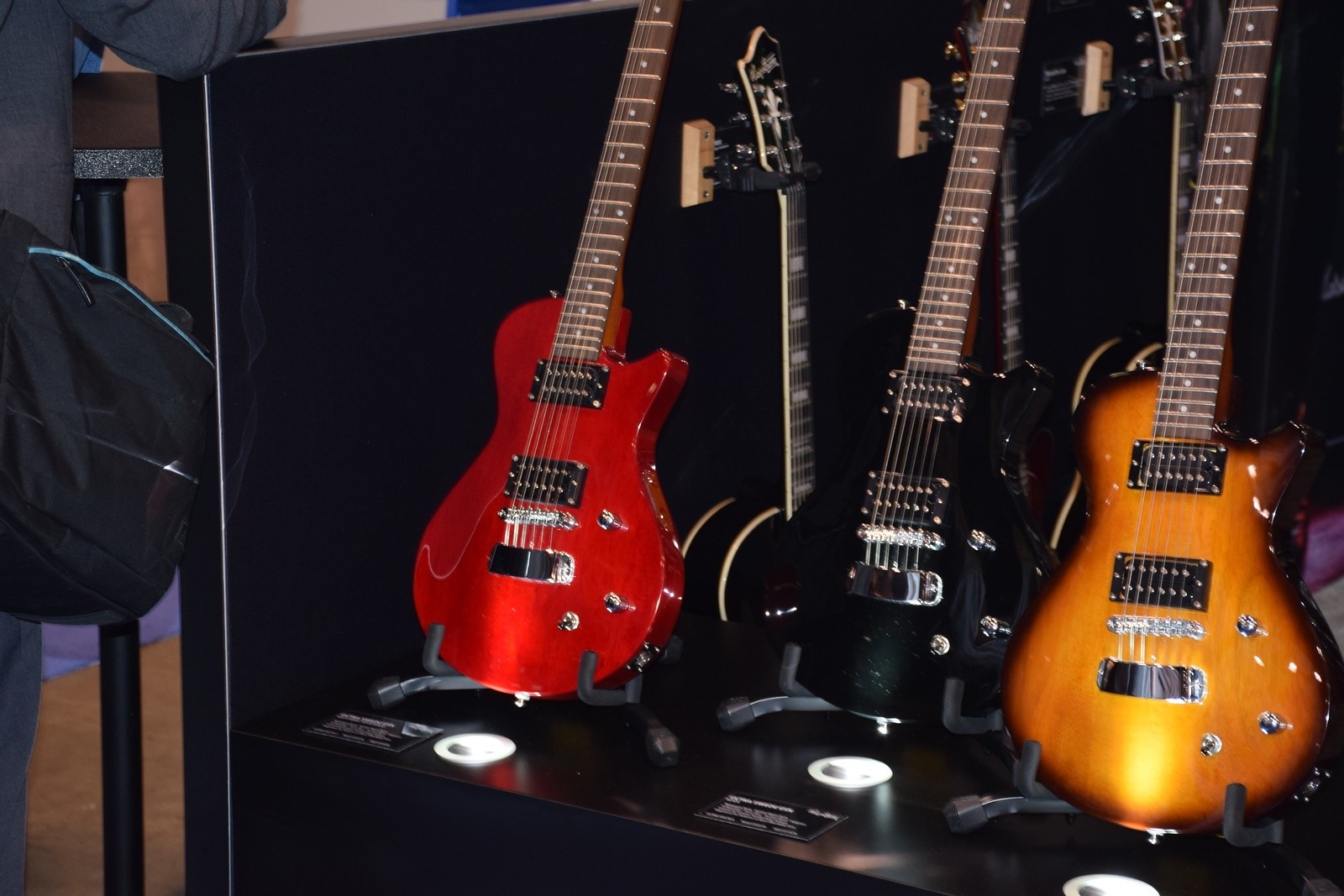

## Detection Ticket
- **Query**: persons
[1,0,287,896]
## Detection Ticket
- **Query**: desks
[64,71,163,896]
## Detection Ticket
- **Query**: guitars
[704,0,1200,712]
[1009,0,1344,832]
[414,0,690,701]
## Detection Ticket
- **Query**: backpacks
[1,209,216,629]
